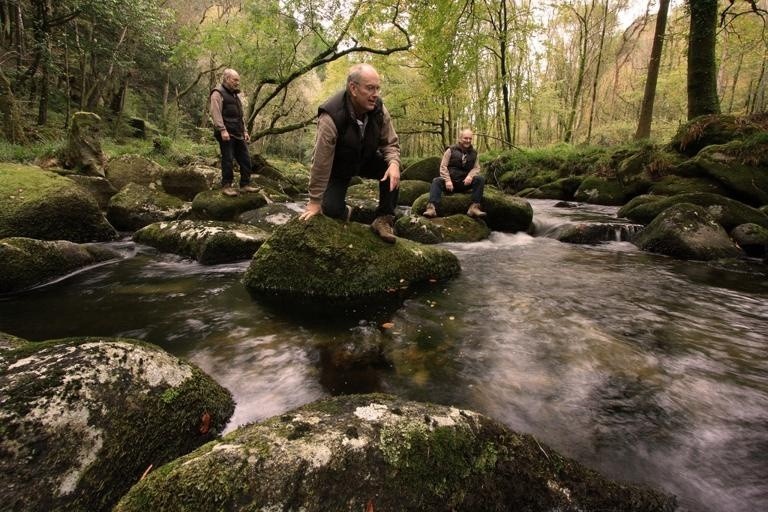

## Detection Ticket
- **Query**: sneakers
[467,202,487,217]
[239,185,261,193]
[222,183,238,196]
[345,205,352,222]
[423,202,437,217]
[371,215,397,244]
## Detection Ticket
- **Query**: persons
[209,67,260,197]
[299,64,401,243]
[423,129,490,219]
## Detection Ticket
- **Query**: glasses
[354,83,382,93]
